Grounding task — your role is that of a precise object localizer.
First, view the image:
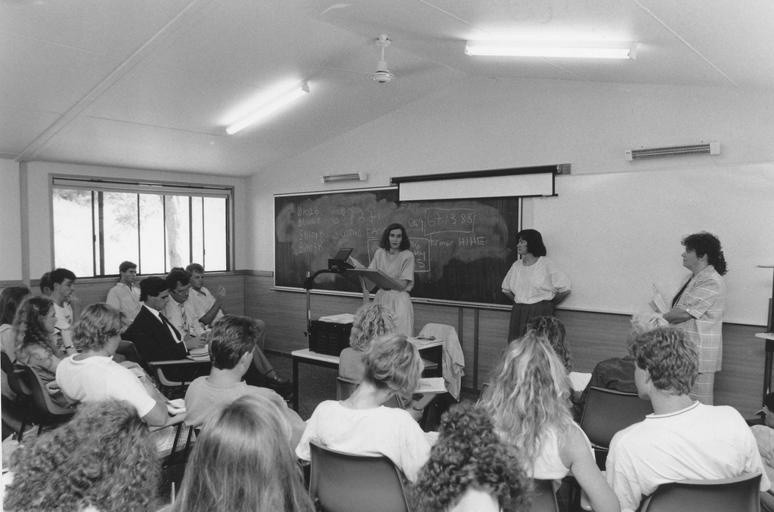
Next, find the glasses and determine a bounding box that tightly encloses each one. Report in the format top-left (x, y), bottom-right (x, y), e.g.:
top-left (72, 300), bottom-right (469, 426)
top-left (463, 45), bottom-right (637, 62)
top-left (321, 171), bottom-right (367, 184)
top-left (624, 141), bottom-right (721, 163)
top-left (225, 82), bottom-right (311, 136)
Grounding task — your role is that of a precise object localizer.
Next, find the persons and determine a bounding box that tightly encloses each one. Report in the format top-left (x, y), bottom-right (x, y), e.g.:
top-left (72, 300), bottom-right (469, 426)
top-left (1, 284), bottom-right (32, 403)
top-left (500, 228), bottom-right (572, 342)
top-left (296, 331), bottom-right (439, 487)
top-left (172, 394), bottom-right (317, 512)
top-left (185, 315), bottom-right (310, 451)
top-left (409, 400), bottom-right (542, 512)
top-left (40, 267), bottom-right (76, 347)
top-left (107, 262), bottom-right (142, 340)
top-left (476, 332), bottom-right (621, 511)
top-left (749, 394), bottom-right (773, 483)
top-left (663, 232), bottom-right (728, 406)
top-left (186, 264), bottom-right (224, 330)
top-left (56, 303), bottom-right (168, 427)
top-left (349, 222), bottom-right (417, 337)
top-left (605, 325), bottom-right (772, 512)
top-left (10, 295), bottom-right (79, 409)
top-left (124, 277), bottom-right (300, 398)
top-left (1, 395), bottom-right (162, 511)
top-left (577, 307), bottom-right (670, 422)
top-left (339, 303), bottom-right (424, 422)
top-left (522, 315), bottom-right (584, 423)
top-left (161, 266), bottom-right (296, 386)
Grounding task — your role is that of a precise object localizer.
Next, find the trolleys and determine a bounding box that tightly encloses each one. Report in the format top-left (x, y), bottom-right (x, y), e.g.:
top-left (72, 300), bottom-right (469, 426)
top-left (303, 34), bottom-right (449, 87)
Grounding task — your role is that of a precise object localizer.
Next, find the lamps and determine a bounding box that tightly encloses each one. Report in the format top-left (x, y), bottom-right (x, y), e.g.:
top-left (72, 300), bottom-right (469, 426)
top-left (321, 171), bottom-right (367, 184)
top-left (624, 141), bottom-right (721, 163)
top-left (225, 82), bottom-right (311, 136)
top-left (463, 45), bottom-right (637, 62)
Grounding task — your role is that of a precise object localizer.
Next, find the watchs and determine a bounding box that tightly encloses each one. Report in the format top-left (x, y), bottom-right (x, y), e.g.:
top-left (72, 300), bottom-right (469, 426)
top-left (65, 344), bottom-right (74, 349)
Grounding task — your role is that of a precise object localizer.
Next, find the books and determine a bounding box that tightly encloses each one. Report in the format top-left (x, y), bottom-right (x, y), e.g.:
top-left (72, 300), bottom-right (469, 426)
top-left (348, 268), bottom-right (405, 290)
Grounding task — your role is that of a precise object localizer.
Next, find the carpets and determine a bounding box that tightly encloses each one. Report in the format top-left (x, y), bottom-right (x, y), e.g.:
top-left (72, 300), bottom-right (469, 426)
top-left (159, 313), bottom-right (183, 341)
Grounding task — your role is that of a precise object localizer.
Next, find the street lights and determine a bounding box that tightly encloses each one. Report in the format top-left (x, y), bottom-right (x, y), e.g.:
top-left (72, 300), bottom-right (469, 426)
top-left (2, 414), bottom-right (46, 469)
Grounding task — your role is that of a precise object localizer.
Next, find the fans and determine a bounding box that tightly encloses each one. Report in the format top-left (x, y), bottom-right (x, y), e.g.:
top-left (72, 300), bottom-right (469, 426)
top-left (271, 184), bottom-right (523, 310)
top-left (303, 34), bottom-right (449, 87)
top-left (518, 163), bottom-right (772, 328)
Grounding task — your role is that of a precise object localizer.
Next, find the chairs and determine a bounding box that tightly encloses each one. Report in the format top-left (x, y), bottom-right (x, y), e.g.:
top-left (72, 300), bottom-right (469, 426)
top-left (0, 321), bottom-right (774, 511)
top-left (270, 374), bottom-right (290, 385)
